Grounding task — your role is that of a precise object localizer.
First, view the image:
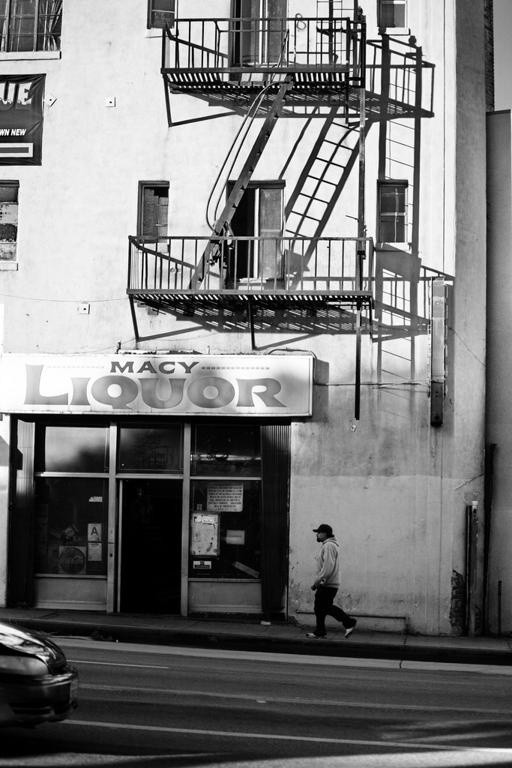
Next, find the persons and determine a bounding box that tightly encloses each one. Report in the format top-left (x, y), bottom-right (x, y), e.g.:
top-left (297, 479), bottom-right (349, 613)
top-left (305, 523), bottom-right (357, 640)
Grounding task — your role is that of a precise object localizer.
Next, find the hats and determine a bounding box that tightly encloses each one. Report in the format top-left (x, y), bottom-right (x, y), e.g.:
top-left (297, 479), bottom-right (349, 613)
top-left (1, 620), bottom-right (76, 728)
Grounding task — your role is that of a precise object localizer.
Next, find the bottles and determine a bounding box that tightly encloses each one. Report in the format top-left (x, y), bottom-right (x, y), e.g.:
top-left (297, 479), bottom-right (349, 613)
top-left (313, 525), bottom-right (332, 533)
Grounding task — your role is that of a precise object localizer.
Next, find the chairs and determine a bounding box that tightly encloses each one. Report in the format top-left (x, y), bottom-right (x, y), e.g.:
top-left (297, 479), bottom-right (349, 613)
top-left (344, 626), bottom-right (356, 638)
top-left (306, 632), bottom-right (327, 639)
